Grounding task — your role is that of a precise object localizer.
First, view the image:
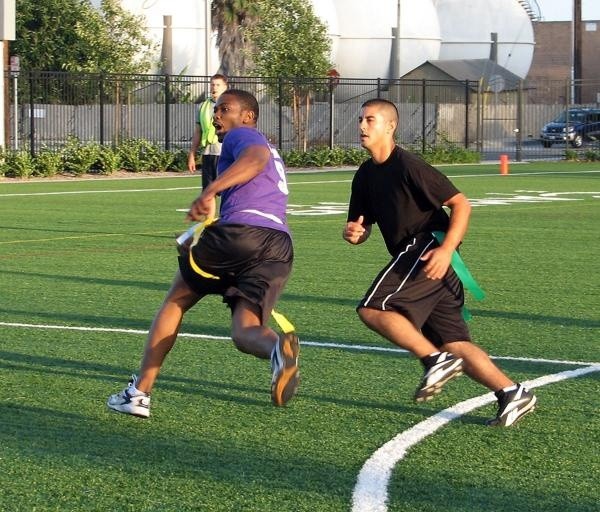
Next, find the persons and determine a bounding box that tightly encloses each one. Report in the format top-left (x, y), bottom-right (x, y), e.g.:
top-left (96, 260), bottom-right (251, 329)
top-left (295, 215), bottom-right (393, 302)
top-left (344, 98), bottom-right (540, 429)
top-left (185, 73), bottom-right (231, 219)
top-left (105, 89), bottom-right (303, 418)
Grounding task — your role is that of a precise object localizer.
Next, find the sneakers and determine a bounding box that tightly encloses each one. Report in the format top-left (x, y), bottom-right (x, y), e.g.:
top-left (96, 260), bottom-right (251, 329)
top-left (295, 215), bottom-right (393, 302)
top-left (487, 382), bottom-right (537, 426)
top-left (415, 351), bottom-right (463, 401)
top-left (270, 332), bottom-right (301, 407)
top-left (106, 388), bottom-right (151, 419)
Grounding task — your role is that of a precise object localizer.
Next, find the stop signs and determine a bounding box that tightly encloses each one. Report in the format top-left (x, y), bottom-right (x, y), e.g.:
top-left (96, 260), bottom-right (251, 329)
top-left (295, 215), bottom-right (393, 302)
top-left (325, 70), bottom-right (340, 89)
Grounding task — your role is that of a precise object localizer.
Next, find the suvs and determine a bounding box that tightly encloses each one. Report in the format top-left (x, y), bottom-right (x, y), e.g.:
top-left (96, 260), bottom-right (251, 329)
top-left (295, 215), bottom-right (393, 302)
top-left (540, 106), bottom-right (600, 149)
